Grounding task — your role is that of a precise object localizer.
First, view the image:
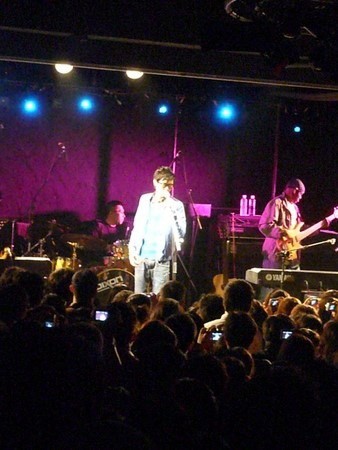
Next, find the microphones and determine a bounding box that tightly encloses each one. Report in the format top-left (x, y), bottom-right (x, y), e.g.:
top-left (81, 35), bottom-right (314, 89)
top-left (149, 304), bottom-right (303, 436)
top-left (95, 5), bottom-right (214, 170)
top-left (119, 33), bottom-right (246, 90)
top-left (221, 226), bottom-right (230, 241)
top-left (176, 150), bottom-right (182, 159)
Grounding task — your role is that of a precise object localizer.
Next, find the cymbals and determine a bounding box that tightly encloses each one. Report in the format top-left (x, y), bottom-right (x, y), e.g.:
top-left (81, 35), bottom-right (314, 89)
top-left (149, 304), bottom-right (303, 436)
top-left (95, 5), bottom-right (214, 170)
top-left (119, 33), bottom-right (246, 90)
top-left (43, 221), bottom-right (71, 232)
top-left (61, 232), bottom-right (107, 249)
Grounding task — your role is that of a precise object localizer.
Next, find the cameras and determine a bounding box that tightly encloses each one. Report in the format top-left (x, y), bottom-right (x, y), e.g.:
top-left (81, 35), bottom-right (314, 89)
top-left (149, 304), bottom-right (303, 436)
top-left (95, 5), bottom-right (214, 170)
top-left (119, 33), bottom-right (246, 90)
top-left (43, 321), bottom-right (55, 328)
top-left (209, 331), bottom-right (223, 341)
top-left (280, 330), bottom-right (292, 339)
top-left (94, 310), bottom-right (109, 321)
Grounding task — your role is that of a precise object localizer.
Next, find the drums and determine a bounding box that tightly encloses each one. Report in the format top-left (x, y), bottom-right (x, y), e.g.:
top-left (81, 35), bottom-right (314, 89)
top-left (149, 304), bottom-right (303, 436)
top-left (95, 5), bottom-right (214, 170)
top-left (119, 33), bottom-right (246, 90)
top-left (104, 256), bottom-right (134, 275)
top-left (88, 265), bottom-right (134, 306)
top-left (113, 239), bottom-right (130, 260)
top-left (51, 255), bottom-right (84, 274)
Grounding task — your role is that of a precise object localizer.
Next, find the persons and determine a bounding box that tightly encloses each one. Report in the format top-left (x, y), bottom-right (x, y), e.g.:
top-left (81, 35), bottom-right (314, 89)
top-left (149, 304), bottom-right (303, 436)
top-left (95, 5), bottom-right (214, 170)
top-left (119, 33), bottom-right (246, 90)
top-left (258, 179), bottom-right (306, 270)
top-left (127, 166), bottom-right (187, 294)
top-left (90, 200), bottom-right (133, 242)
top-left (0, 266), bottom-right (337, 450)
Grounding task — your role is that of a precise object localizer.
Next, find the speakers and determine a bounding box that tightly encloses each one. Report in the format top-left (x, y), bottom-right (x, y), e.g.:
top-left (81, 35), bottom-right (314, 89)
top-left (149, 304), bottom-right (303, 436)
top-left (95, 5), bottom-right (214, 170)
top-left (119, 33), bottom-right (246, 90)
top-left (245, 267), bottom-right (338, 302)
top-left (14, 256), bottom-right (52, 278)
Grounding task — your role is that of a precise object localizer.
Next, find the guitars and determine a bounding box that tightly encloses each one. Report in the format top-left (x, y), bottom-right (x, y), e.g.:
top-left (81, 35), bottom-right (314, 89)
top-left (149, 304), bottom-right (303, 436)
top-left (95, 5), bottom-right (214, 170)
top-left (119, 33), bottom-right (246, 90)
top-left (276, 206), bottom-right (338, 260)
top-left (212, 213), bottom-right (235, 295)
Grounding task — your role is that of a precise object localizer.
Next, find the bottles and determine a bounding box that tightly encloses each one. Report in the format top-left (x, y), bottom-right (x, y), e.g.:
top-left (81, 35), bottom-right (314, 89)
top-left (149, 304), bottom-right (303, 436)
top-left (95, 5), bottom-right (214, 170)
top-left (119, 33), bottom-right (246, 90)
top-left (248, 195), bottom-right (256, 217)
top-left (240, 195), bottom-right (248, 216)
